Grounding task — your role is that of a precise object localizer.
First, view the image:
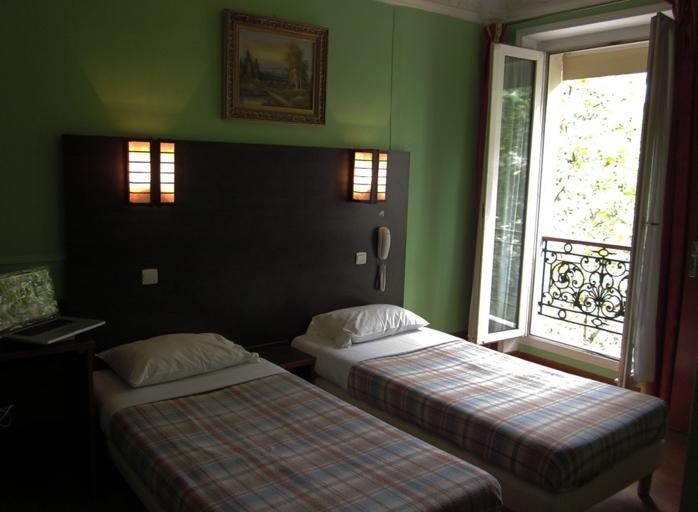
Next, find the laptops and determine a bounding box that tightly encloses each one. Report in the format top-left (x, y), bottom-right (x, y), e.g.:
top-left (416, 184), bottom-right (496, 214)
top-left (0, 265), bottom-right (108, 346)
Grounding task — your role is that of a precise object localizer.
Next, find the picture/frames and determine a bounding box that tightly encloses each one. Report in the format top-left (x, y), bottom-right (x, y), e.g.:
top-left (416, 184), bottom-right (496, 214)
top-left (220, 8), bottom-right (327, 127)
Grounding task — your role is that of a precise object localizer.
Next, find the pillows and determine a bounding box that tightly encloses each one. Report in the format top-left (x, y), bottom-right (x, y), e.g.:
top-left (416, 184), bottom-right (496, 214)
top-left (305, 303), bottom-right (431, 350)
top-left (94, 333), bottom-right (260, 389)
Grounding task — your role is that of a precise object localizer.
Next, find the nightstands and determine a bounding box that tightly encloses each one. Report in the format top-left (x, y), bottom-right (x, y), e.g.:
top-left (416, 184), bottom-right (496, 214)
top-left (0, 327), bottom-right (95, 433)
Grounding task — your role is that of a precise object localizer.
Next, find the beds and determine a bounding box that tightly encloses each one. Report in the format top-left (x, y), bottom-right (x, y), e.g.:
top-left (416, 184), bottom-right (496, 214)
top-left (84, 348), bottom-right (503, 512)
top-left (291, 313), bottom-right (669, 511)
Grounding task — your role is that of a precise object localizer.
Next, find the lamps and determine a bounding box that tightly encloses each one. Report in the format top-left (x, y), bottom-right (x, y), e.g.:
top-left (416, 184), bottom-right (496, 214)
top-left (121, 138), bottom-right (180, 209)
top-left (347, 148), bottom-right (388, 204)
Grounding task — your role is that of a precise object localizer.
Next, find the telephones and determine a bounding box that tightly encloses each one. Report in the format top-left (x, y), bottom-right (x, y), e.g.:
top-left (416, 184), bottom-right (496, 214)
top-left (378, 226), bottom-right (390, 293)
